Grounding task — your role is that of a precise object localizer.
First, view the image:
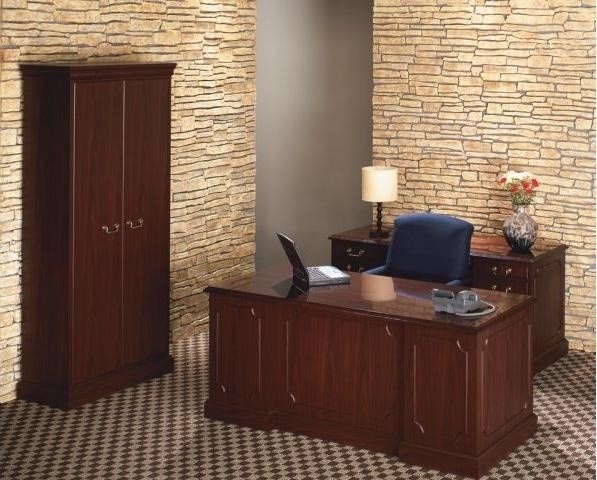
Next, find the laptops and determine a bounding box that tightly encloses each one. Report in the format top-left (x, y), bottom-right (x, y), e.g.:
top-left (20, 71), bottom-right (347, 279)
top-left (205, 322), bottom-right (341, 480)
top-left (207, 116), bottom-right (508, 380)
top-left (276, 232), bottom-right (351, 287)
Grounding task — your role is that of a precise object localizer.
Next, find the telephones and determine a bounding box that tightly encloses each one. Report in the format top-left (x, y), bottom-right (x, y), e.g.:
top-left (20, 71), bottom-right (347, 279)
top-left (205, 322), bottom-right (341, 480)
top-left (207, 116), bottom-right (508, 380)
top-left (432, 288), bottom-right (485, 314)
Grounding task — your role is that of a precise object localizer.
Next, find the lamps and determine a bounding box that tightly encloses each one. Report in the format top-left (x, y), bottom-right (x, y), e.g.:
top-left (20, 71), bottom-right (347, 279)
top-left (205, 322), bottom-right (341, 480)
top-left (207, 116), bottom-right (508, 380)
top-left (361, 157), bottom-right (400, 239)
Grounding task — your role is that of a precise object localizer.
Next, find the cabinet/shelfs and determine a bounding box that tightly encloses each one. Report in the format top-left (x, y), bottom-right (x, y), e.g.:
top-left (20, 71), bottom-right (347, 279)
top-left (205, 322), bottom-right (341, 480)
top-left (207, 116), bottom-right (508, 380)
top-left (15, 61), bottom-right (181, 412)
top-left (202, 266), bottom-right (540, 480)
top-left (327, 222), bottom-right (572, 375)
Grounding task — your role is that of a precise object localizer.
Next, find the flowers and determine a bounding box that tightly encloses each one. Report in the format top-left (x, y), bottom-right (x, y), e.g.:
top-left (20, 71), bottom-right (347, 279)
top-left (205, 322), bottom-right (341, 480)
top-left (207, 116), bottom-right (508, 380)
top-left (497, 170), bottom-right (541, 206)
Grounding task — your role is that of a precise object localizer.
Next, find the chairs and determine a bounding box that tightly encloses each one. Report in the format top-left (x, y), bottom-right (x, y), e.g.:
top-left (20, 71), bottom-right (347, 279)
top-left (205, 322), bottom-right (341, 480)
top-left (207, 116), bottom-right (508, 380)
top-left (360, 212), bottom-right (477, 286)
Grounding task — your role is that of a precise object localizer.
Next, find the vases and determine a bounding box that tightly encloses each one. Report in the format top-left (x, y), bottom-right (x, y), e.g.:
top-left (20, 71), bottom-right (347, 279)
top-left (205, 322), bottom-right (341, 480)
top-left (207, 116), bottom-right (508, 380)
top-left (501, 202), bottom-right (538, 253)
